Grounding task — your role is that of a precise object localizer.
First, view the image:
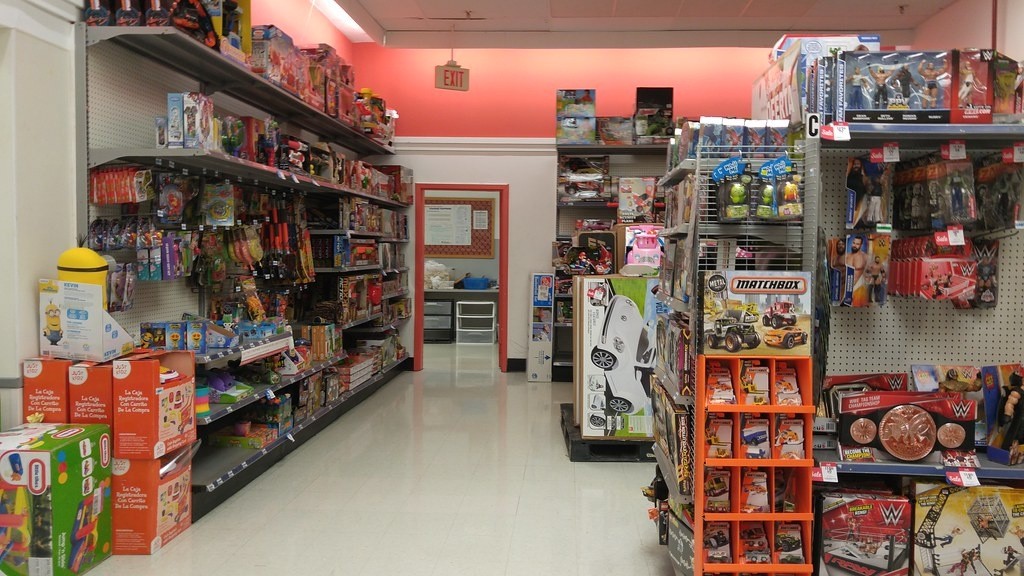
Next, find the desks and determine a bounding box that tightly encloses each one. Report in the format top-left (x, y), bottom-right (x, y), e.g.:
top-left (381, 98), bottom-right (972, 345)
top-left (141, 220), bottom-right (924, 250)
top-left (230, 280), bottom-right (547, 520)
top-left (423, 289), bottom-right (500, 345)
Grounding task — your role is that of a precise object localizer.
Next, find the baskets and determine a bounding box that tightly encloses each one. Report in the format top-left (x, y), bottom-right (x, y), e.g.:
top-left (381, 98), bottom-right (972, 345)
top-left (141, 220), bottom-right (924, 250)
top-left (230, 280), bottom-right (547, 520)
top-left (464, 278), bottom-right (488, 289)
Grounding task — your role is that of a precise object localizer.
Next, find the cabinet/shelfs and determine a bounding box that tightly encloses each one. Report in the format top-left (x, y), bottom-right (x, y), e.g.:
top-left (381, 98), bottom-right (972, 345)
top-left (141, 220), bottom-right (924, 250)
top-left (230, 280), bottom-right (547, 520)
top-left (548, 144), bottom-right (672, 379)
top-left (813, 123), bottom-right (1024, 576)
top-left (73, 21), bottom-right (411, 526)
top-left (651, 149), bottom-right (815, 575)
top-left (423, 299), bottom-right (496, 345)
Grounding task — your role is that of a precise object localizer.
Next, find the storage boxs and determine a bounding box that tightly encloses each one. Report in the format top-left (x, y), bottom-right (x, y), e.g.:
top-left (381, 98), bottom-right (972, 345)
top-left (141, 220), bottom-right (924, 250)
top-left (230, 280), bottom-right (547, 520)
top-left (558, 154), bottom-right (657, 222)
top-left (434, 281), bottom-right (455, 290)
top-left (649, 173), bottom-right (693, 498)
top-left (21, 346), bottom-right (198, 555)
top-left (166, 92), bottom-right (214, 149)
top-left (137, 164), bottom-right (413, 451)
top-left (250, 25), bottom-right (357, 127)
top-left (552, 87), bottom-right (679, 145)
top-left (768, 33), bottom-right (1024, 113)
top-left (239, 116), bottom-right (266, 163)
top-left (825, 361), bottom-right (1024, 466)
top-left (812, 476), bottom-right (1024, 576)
top-left (462, 278), bottom-right (488, 289)
top-left (569, 274), bottom-right (660, 442)
top-left (0, 424), bottom-right (116, 576)
